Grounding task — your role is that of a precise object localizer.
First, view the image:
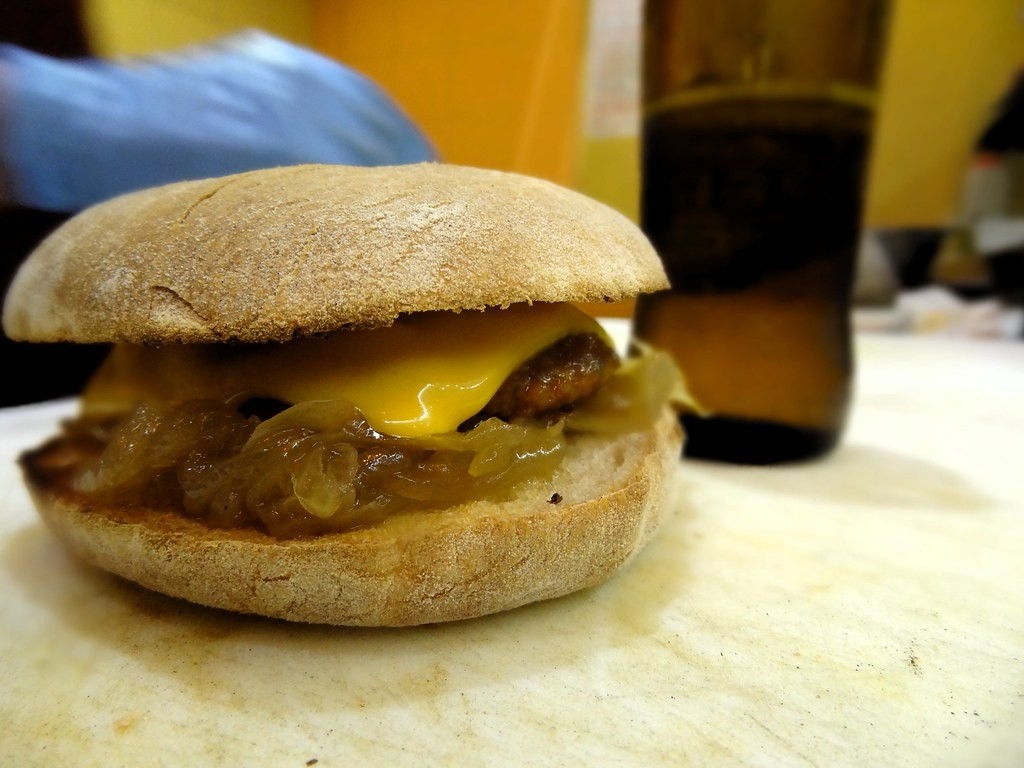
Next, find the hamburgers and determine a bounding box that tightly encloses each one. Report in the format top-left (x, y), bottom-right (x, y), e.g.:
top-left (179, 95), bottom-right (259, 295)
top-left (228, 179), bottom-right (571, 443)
top-left (3, 158), bottom-right (713, 627)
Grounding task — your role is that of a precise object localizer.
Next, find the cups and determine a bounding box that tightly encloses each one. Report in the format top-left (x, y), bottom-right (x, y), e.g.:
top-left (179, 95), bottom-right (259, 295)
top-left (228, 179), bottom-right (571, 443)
top-left (628, 0), bottom-right (895, 466)
